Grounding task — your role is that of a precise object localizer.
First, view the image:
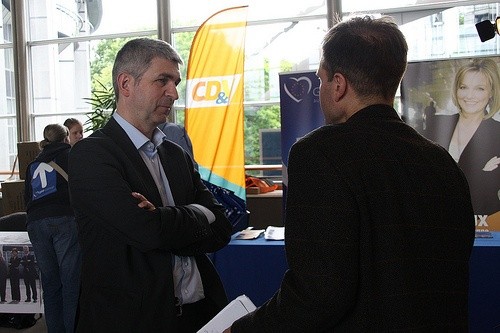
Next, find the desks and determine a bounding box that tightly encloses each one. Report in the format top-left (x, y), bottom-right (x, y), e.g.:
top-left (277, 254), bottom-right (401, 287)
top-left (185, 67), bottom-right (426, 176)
top-left (468, 230), bottom-right (500, 333)
top-left (206, 232), bottom-right (287, 304)
top-left (244, 190), bottom-right (285, 230)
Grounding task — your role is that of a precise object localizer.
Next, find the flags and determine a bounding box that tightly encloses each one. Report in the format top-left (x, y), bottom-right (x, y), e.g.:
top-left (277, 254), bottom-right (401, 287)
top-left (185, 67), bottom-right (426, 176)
top-left (186, 8), bottom-right (249, 205)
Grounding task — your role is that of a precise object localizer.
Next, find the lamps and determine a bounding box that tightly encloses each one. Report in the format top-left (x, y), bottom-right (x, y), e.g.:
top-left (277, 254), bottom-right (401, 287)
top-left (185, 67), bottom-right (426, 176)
top-left (474, 16), bottom-right (500, 43)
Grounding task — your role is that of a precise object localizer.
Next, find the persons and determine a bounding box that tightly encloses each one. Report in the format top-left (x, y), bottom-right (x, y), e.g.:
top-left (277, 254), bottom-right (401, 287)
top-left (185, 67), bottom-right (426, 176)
top-left (25, 118), bottom-right (83, 333)
top-left (221, 17), bottom-right (476, 333)
top-left (70, 37), bottom-right (232, 333)
top-left (0, 244), bottom-right (39, 305)
top-left (413, 58), bottom-right (500, 222)
